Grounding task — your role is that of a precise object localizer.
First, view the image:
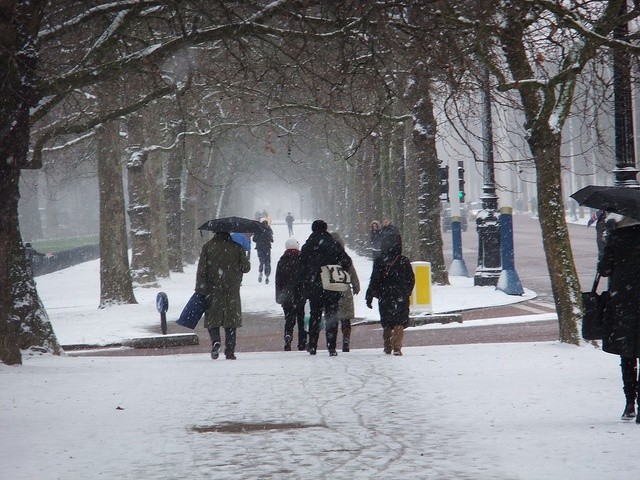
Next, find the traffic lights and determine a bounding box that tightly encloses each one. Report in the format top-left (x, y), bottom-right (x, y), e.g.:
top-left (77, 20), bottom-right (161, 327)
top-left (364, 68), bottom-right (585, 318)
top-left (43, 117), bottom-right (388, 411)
top-left (459, 166), bottom-right (465, 203)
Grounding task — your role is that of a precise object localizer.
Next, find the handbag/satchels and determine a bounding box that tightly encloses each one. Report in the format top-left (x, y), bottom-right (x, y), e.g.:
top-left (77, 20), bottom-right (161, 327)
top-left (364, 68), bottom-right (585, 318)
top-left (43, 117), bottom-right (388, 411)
top-left (175, 291), bottom-right (211, 330)
top-left (320, 264), bottom-right (351, 292)
top-left (582, 261), bottom-right (612, 339)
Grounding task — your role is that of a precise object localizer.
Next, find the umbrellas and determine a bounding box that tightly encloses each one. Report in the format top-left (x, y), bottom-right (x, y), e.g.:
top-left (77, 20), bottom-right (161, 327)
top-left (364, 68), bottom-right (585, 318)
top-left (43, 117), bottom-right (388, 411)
top-left (568, 185), bottom-right (640, 221)
top-left (196, 215), bottom-right (267, 238)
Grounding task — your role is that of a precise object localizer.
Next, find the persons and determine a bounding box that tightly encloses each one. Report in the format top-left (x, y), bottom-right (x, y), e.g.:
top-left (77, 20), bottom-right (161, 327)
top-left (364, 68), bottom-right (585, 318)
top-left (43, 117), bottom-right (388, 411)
top-left (195, 229), bottom-right (250, 360)
top-left (285, 212), bottom-right (295, 238)
top-left (364, 234), bottom-right (416, 356)
top-left (252, 220), bottom-right (274, 284)
top-left (230, 232), bottom-right (251, 286)
top-left (369, 220), bottom-right (382, 262)
top-left (330, 231), bottom-right (361, 352)
top-left (261, 209), bottom-right (269, 219)
top-left (580, 218), bottom-right (640, 424)
top-left (300, 219), bottom-right (352, 356)
top-left (254, 210), bottom-right (261, 220)
top-left (586, 209), bottom-right (610, 258)
top-left (373, 217), bottom-right (402, 254)
top-left (275, 238), bottom-right (308, 351)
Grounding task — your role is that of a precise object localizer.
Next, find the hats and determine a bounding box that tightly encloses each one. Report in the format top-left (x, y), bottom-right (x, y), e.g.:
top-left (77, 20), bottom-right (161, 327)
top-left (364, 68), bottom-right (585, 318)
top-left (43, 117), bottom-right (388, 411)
top-left (312, 220), bottom-right (327, 231)
top-left (285, 238), bottom-right (299, 249)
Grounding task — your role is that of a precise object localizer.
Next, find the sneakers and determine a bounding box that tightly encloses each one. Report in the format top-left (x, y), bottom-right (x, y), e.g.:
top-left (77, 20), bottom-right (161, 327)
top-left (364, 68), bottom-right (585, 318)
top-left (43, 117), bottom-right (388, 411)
top-left (393, 349), bottom-right (401, 355)
top-left (620, 404), bottom-right (636, 420)
top-left (211, 342), bottom-right (221, 359)
top-left (329, 349), bottom-right (336, 356)
top-left (283, 334), bottom-right (292, 351)
top-left (226, 351), bottom-right (237, 359)
top-left (258, 273), bottom-right (263, 282)
top-left (265, 280), bottom-right (270, 284)
